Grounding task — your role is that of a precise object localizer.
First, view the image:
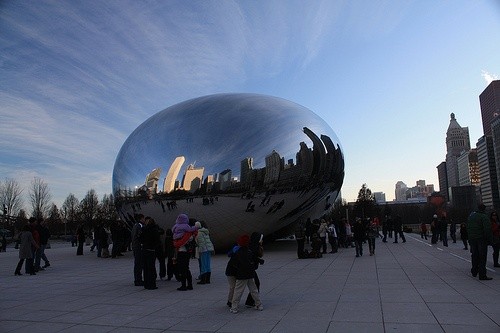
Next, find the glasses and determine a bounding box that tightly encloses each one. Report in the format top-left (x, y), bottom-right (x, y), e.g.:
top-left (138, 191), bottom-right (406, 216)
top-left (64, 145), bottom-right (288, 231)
top-left (492, 213), bottom-right (497, 216)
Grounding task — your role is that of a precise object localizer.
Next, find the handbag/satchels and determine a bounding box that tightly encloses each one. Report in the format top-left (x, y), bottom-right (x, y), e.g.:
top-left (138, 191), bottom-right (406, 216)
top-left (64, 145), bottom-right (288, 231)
top-left (101, 248), bottom-right (109, 257)
top-left (195, 246), bottom-right (199, 260)
top-left (431, 220), bottom-right (436, 228)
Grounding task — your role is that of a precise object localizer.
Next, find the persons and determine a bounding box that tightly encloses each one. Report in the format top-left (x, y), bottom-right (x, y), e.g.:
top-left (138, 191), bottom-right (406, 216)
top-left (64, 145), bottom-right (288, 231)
top-left (466, 203), bottom-right (500, 280)
top-left (420, 215), bottom-right (456, 247)
top-left (131, 212), bottom-right (214, 291)
top-left (240, 191), bottom-right (285, 215)
top-left (161, 200), bottom-right (176, 213)
top-left (203, 195), bottom-right (218, 205)
top-left (460, 223), bottom-right (468, 250)
top-left (186, 197), bottom-right (193, 203)
top-left (15, 215), bottom-right (50, 275)
top-left (295, 215), bottom-right (406, 258)
top-left (225, 231), bottom-right (265, 314)
top-left (71, 218), bottom-right (132, 258)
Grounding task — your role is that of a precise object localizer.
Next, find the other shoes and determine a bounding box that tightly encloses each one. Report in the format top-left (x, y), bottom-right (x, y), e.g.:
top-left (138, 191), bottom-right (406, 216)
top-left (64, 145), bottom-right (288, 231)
top-left (227, 302), bottom-right (232, 307)
top-left (402, 240), bottom-right (406, 243)
top-left (230, 309), bottom-right (237, 313)
top-left (135, 281), bottom-right (144, 286)
top-left (177, 287), bottom-right (185, 291)
top-left (330, 251), bottom-right (337, 253)
top-left (168, 276), bottom-right (171, 280)
top-left (393, 241), bottom-right (398, 243)
top-left (186, 286), bottom-right (193, 290)
top-left (245, 304), bottom-right (256, 308)
top-left (254, 305), bottom-right (263, 310)
top-left (14, 263), bottom-right (50, 276)
top-left (472, 273), bottom-right (477, 276)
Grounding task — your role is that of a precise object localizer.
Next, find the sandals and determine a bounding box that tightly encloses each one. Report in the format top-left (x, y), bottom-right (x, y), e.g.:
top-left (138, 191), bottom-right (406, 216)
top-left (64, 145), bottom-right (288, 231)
top-left (481, 277), bottom-right (492, 280)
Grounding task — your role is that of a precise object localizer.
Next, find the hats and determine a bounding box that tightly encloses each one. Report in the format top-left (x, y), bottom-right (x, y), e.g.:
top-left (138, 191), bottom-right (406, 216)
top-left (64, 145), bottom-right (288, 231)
top-left (239, 234), bottom-right (249, 244)
top-left (433, 214), bottom-right (437, 219)
top-left (195, 221), bottom-right (201, 228)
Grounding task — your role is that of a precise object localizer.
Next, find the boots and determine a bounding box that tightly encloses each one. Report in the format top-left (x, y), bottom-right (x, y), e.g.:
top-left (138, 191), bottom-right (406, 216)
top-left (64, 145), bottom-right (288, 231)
top-left (206, 272), bottom-right (211, 283)
top-left (321, 246), bottom-right (327, 253)
top-left (197, 273), bottom-right (206, 284)
top-left (493, 253), bottom-right (500, 267)
